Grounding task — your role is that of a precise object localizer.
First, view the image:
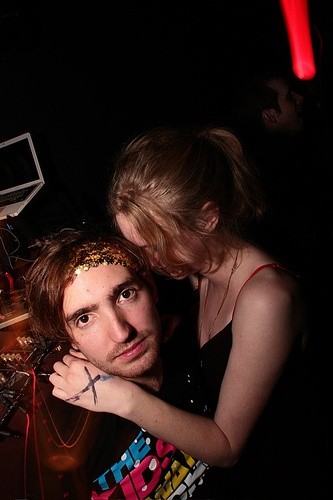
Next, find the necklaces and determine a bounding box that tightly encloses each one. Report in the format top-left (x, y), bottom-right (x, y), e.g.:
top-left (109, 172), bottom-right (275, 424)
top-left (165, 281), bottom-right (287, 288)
top-left (202, 241), bottom-right (237, 343)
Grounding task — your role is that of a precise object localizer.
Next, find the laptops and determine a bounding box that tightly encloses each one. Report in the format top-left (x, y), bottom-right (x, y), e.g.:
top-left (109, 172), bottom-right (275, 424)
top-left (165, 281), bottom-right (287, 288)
top-left (0, 134), bottom-right (46, 219)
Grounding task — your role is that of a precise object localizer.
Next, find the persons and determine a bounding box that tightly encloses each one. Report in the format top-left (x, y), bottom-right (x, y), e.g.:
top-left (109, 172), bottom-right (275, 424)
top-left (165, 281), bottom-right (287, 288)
top-left (41, 120), bottom-right (313, 500)
top-left (16, 229), bottom-right (218, 498)
